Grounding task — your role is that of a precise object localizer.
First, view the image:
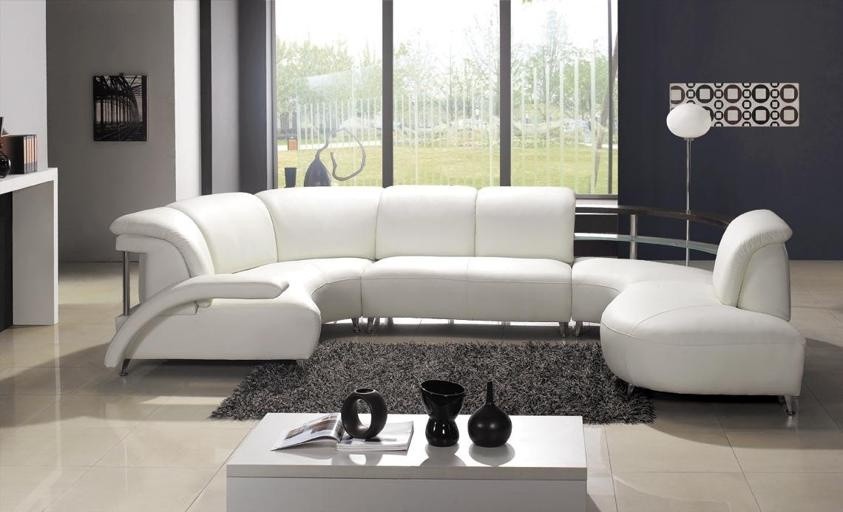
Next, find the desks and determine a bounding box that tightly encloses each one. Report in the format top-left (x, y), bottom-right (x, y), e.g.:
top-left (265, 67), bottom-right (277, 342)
top-left (0, 167), bottom-right (58, 331)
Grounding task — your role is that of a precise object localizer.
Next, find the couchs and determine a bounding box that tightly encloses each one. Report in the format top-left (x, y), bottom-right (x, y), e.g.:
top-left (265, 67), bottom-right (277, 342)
top-left (571, 209), bottom-right (807, 414)
top-left (103, 184), bottom-right (576, 378)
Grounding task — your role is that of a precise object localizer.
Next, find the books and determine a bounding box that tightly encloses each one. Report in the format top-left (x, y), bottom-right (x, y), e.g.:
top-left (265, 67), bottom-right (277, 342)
top-left (2, 133), bottom-right (38, 174)
top-left (271, 414), bottom-right (414, 451)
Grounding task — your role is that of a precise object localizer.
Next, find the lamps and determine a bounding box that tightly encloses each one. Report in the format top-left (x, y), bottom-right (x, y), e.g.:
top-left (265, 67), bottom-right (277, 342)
top-left (665, 103), bottom-right (711, 266)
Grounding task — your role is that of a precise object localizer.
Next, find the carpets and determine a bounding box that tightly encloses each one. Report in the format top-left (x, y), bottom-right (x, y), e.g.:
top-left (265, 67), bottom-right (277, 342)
top-left (208, 340), bottom-right (655, 424)
